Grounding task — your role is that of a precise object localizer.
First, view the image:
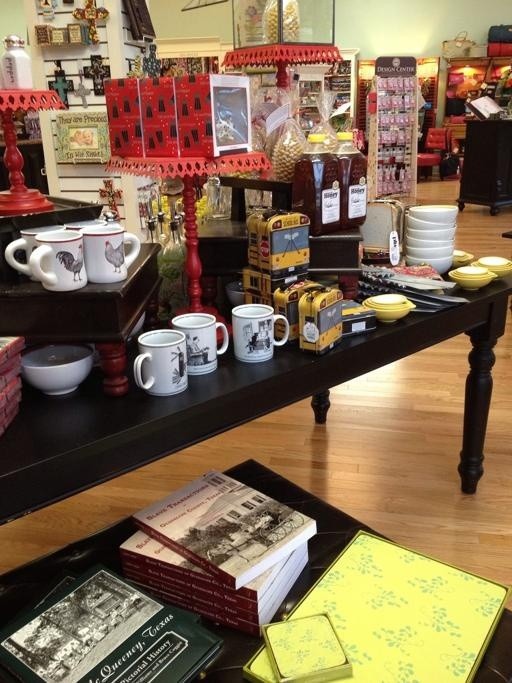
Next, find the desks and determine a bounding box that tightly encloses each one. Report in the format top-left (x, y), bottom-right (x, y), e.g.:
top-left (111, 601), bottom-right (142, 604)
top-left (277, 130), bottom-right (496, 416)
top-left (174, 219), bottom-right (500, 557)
top-left (0, 263), bottom-right (510, 523)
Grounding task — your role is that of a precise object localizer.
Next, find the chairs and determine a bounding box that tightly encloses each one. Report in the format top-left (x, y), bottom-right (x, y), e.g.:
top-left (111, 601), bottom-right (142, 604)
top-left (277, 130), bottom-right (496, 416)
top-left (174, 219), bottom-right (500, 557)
top-left (418, 125), bottom-right (452, 182)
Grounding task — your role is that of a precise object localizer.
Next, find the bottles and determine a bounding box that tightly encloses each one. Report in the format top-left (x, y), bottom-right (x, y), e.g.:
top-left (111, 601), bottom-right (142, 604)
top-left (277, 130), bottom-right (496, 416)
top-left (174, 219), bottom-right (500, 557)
top-left (144, 212), bottom-right (190, 319)
top-left (332, 132), bottom-right (366, 228)
top-left (2, 34), bottom-right (33, 90)
top-left (291, 133), bottom-right (341, 236)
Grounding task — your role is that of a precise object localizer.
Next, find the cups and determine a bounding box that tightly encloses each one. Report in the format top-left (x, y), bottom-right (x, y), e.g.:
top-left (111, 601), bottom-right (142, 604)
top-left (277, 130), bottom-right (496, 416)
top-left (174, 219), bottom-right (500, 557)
top-left (134, 327), bottom-right (191, 397)
top-left (171, 311), bottom-right (231, 377)
top-left (4, 220), bottom-right (141, 291)
top-left (232, 303), bottom-right (291, 365)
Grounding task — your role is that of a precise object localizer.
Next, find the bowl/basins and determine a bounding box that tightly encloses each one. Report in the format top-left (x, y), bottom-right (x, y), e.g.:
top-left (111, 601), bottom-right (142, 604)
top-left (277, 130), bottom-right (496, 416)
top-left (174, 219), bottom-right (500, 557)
top-left (451, 249), bottom-right (512, 293)
top-left (406, 204), bottom-right (459, 274)
top-left (364, 294), bottom-right (416, 322)
top-left (21, 344), bottom-right (94, 397)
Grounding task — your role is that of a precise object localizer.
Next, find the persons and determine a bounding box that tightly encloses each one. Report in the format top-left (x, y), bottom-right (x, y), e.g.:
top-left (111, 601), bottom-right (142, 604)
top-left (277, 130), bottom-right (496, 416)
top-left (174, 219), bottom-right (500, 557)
top-left (70, 130), bottom-right (84, 149)
top-left (82, 129), bottom-right (98, 148)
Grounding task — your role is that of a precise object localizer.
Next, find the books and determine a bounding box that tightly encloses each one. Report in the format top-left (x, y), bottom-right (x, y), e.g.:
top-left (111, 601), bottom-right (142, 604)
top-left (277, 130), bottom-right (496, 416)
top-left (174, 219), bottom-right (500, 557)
top-left (119, 467), bottom-right (319, 639)
top-left (0, 569), bottom-right (227, 683)
